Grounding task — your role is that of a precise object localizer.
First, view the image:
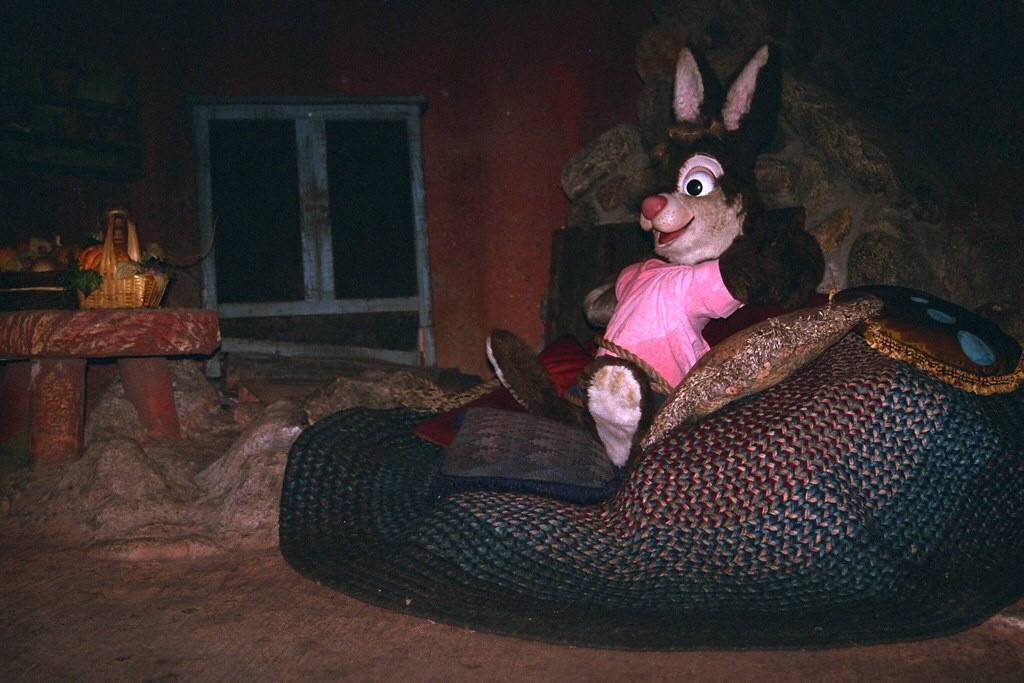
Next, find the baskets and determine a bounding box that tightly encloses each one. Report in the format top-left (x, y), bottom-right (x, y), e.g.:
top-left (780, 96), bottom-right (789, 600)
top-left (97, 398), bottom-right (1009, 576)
top-left (76, 211), bottom-right (167, 309)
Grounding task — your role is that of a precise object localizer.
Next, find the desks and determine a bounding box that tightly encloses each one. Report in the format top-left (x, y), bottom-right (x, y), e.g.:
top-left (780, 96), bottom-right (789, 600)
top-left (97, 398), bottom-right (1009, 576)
top-left (0, 307), bottom-right (220, 471)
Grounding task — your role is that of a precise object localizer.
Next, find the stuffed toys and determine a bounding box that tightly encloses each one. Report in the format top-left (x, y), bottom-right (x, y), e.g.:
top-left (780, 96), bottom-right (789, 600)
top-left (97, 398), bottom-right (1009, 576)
top-left (484, 44), bottom-right (825, 474)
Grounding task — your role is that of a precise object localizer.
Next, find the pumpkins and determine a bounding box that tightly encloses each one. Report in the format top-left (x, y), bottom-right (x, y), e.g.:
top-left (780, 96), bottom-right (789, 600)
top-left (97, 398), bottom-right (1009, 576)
top-left (78, 244), bottom-right (133, 276)
